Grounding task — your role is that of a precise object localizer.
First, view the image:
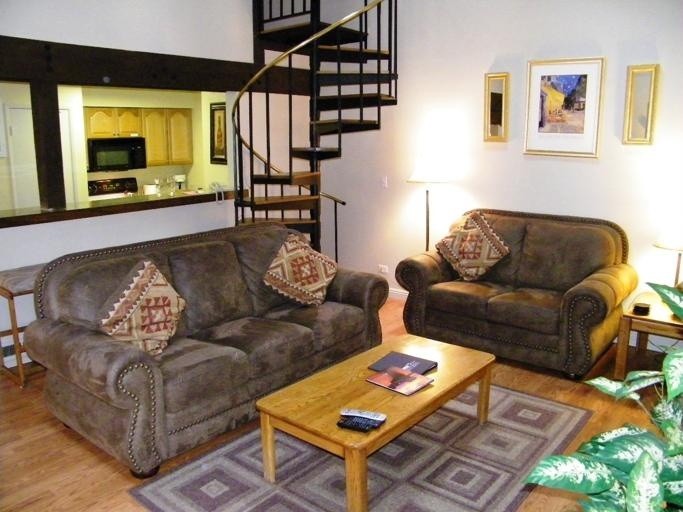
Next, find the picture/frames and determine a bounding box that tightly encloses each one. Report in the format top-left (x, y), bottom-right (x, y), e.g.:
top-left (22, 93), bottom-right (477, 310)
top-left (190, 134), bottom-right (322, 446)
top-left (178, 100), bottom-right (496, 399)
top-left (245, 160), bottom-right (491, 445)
top-left (521, 59), bottom-right (606, 158)
top-left (209, 102), bottom-right (227, 164)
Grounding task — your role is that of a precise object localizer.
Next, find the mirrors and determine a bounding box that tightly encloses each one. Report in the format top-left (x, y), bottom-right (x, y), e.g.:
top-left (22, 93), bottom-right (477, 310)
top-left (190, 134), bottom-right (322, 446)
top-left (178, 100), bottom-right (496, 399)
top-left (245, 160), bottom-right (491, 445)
top-left (486, 72), bottom-right (508, 143)
top-left (623, 65), bottom-right (661, 144)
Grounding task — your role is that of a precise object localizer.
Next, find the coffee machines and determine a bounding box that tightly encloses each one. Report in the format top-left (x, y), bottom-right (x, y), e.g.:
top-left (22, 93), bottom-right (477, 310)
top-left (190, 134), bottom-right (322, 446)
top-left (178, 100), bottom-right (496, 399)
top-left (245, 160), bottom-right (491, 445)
top-left (174, 175), bottom-right (185, 190)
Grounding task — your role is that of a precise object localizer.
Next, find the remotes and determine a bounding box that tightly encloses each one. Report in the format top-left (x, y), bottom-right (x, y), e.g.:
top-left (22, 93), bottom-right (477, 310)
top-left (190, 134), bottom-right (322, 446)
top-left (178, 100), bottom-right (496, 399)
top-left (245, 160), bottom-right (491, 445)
top-left (340, 408), bottom-right (385, 422)
top-left (337, 419), bottom-right (369, 432)
top-left (346, 417), bottom-right (379, 426)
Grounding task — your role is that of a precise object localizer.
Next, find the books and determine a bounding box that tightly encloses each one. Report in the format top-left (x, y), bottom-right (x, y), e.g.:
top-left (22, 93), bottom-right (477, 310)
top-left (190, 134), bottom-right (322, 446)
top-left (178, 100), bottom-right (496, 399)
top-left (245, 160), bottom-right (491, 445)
top-left (368, 365), bottom-right (435, 395)
top-left (368, 350), bottom-right (437, 375)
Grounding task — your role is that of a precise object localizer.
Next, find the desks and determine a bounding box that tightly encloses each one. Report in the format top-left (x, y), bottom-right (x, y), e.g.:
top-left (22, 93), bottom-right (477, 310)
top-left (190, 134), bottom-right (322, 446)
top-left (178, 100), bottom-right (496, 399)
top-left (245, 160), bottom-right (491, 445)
top-left (0, 264), bottom-right (55, 388)
top-left (613, 292), bottom-right (683, 381)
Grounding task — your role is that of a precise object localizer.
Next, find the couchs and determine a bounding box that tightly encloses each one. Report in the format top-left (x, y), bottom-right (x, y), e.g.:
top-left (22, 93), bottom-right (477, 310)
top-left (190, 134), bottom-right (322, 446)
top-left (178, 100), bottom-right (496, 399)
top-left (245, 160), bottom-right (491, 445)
top-left (21, 221), bottom-right (390, 479)
top-left (393, 209), bottom-right (639, 382)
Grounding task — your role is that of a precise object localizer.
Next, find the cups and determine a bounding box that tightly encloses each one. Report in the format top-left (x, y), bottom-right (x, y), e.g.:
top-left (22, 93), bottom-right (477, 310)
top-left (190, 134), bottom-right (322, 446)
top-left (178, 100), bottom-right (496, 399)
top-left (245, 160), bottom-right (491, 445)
top-left (155, 175), bottom-right (163, 197)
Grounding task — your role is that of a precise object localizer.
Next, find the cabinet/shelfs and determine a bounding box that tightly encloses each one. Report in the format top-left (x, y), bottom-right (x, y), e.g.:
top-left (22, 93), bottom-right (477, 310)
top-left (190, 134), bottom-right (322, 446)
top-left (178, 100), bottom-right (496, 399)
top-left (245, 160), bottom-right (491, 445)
top-left (83, 105), bottom-right (195, 165)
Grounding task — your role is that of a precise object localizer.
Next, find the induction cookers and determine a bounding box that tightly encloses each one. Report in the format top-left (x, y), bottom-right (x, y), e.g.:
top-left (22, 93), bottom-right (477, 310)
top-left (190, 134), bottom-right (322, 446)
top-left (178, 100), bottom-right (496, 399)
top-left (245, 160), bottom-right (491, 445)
top-left (88, 177), bottom-right (138, 202)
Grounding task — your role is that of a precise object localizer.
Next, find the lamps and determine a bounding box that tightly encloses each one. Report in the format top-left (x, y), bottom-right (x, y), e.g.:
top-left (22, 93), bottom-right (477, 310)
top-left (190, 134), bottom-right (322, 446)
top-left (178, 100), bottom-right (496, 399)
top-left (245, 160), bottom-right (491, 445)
top-left (408, 155), bottom-right (449, 251)
top-left (652, 234), bottom-right (682, 304)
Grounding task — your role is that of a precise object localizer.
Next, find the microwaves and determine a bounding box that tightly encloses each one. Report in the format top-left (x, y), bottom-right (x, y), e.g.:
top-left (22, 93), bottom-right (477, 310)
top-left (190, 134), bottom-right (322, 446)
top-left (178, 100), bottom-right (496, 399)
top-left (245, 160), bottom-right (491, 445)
top-left (86, 137), bottom-right (147, 173)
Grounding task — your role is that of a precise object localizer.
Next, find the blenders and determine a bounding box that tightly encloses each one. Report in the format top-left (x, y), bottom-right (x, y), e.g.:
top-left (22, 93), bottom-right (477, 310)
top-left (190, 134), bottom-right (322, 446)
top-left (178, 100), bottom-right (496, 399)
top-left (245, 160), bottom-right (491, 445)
top-left (142, 184), bottom-right (156, 195)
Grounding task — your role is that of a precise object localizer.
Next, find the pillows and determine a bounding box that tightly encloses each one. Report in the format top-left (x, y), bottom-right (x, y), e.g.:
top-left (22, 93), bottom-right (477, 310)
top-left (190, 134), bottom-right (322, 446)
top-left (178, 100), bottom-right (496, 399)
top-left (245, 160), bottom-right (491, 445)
top-left (96, 259), bottom-right (189, 359)
top-left (264, 234), bottom-right (340, 306)
top-left (434, 211), bottom-right (507, 282)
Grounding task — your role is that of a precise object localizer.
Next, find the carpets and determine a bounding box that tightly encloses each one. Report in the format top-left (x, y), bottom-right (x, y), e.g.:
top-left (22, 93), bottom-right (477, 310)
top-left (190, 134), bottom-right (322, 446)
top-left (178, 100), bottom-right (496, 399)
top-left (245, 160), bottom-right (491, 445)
top-left (128, 382), bottom-right (595, 512)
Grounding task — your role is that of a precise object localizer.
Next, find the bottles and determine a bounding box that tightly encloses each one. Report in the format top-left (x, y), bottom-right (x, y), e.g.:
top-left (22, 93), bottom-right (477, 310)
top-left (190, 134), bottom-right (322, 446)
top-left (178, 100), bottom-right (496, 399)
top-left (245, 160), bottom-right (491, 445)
top-left (217, 116), bottom-right (223, 150)
top-left (167, 175), bottom-right (176, 196)
